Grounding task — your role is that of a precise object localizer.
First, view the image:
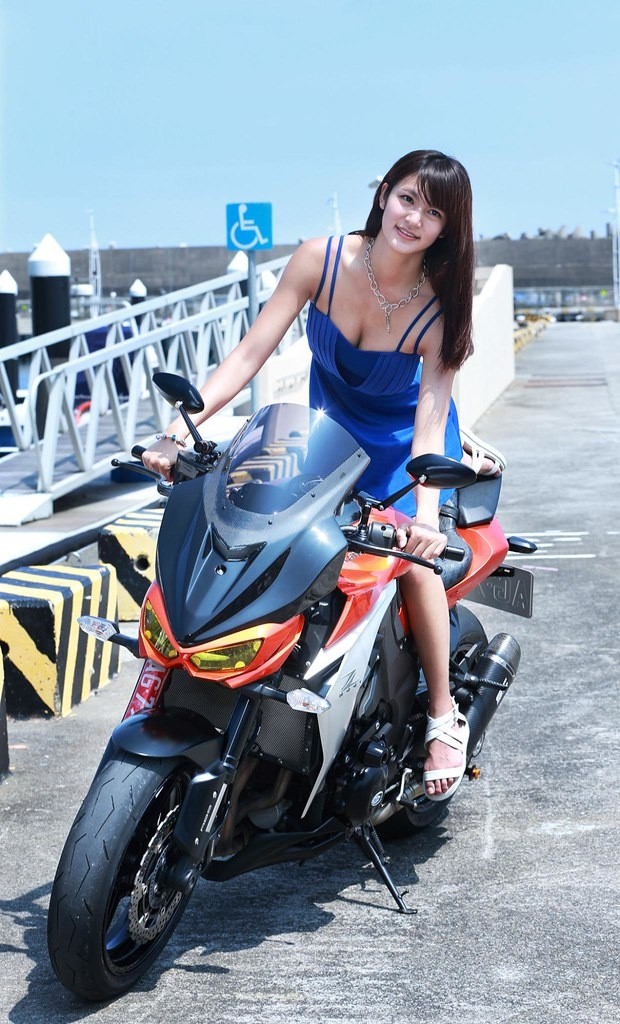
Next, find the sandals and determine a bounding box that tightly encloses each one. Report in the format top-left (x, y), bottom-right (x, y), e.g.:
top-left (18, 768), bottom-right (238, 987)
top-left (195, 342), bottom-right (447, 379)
top-left (459, 424), bottom-right (507, 477)
top-left (422, 696), bottom-right (470, 802)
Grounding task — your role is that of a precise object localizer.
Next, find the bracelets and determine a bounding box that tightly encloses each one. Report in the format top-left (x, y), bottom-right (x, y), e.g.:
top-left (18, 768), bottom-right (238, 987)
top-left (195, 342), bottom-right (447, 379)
top-left (155, 434), bottom-right (186, 448)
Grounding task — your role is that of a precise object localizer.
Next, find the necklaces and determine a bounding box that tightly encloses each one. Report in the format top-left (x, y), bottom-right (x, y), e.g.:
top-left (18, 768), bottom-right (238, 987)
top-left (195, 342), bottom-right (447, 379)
top-left (364, 236), bottom-right (428, 334)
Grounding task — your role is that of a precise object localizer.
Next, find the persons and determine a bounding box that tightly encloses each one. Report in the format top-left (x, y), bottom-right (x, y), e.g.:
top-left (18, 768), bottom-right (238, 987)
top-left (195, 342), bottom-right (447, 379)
top-left (140, 148), bottom-right (507, 802)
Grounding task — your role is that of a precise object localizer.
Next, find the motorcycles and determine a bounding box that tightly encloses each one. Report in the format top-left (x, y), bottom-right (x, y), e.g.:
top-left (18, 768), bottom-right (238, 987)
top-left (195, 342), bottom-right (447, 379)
top-left (46, 371), bottom-right (537, 1001)
